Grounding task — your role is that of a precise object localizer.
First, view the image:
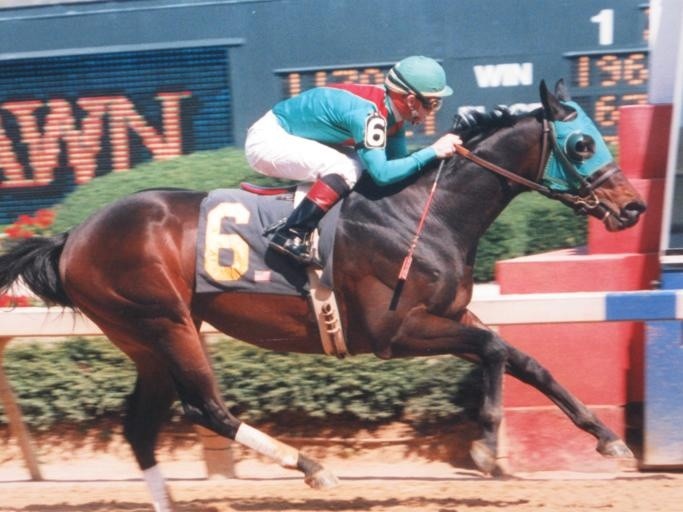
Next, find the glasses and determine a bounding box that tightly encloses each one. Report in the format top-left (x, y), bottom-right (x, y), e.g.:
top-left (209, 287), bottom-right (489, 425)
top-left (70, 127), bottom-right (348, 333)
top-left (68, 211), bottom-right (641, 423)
top-left (387, 64), bottom-right (442, 114)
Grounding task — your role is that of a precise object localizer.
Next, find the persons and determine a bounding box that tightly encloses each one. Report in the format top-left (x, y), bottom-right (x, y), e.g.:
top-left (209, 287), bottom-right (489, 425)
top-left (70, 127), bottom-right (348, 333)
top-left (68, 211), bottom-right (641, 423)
top-left (245, 57), bottom-right (463, 269)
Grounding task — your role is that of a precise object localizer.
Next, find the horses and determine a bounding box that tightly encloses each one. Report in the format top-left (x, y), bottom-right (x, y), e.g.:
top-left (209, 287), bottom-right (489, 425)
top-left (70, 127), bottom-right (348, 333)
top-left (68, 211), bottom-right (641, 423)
top-left (0, 79), bottom-right (646, 512)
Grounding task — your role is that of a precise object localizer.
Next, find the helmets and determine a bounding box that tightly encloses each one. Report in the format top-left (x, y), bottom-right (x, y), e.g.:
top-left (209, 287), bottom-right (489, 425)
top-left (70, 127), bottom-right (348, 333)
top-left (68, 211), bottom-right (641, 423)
top-left (385, 56), bottom-right (455, 98)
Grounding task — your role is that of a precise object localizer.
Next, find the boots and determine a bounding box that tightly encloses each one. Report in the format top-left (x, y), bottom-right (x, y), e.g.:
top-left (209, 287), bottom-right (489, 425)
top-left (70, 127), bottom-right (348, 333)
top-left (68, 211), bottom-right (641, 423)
top-left (268, 173), bottom-right (351, 269)
